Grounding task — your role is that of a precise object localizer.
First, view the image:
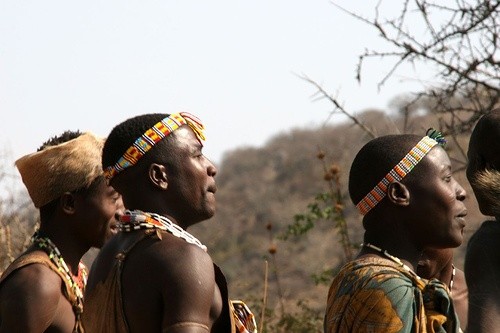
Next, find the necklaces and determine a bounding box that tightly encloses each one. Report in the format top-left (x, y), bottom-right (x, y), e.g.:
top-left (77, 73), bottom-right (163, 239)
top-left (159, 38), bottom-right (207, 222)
top-left (360, 243), bottom-right (456, 295)
top-left (111, 210), bottom-right (208, 252)
top-left (29, 228), bottom-right (88, 313)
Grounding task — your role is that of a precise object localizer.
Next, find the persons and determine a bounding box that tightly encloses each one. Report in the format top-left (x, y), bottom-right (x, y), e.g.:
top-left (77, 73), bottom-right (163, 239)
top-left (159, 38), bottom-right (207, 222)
top-left (464, 112), bottom-right (500, 333)
top-left (83, 114), bottom-right (258, 333)
top-left (0, 132), bottom-right (128, 333)
top-left (323, 128), bottom-right (467, 333)
